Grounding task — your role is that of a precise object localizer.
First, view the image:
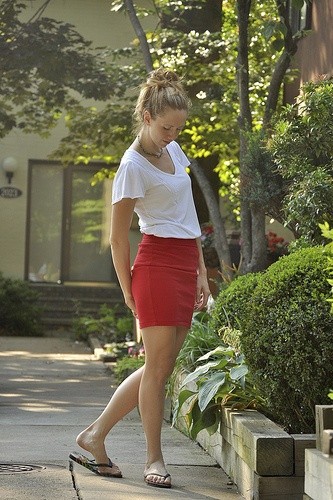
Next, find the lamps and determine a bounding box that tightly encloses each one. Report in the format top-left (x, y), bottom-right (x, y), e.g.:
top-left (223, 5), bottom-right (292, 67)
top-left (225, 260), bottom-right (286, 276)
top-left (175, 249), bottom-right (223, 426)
top-left (3, 157), bottom-right (19, 184)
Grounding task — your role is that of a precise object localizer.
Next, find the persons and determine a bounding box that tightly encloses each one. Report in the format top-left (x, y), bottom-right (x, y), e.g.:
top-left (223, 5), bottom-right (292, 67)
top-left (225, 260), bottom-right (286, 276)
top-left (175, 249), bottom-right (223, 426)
top-left (70, 70), bottom-right (210, 487)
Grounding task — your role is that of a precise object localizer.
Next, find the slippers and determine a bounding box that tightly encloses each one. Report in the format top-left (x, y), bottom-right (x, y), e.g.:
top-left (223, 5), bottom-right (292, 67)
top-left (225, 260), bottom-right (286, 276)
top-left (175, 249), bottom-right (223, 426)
top-left (143, 473), bottom-right (171, 488)
top-left (68, 450), bottom-right (122, 478)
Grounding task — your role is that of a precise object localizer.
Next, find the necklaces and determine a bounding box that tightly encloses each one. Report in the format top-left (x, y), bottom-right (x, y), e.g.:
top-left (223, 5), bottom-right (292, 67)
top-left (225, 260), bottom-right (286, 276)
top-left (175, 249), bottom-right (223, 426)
top-left (137, 135), bottom-right (163, 158)
